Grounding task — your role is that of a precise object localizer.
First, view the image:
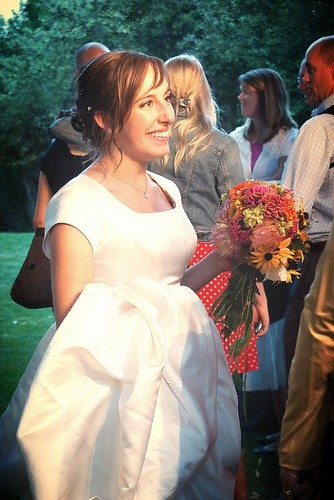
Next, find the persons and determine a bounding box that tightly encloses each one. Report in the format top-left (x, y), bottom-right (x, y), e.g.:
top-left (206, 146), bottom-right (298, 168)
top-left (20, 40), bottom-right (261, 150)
top-left (42, 49), bottom-right (269, 500)
top-left (32, 36), bottom-right (334, 500)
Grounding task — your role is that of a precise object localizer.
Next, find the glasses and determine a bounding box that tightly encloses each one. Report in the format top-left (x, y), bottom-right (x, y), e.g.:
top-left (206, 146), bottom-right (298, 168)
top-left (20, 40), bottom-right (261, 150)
top-left (297, 76), bottom-right (303, 82)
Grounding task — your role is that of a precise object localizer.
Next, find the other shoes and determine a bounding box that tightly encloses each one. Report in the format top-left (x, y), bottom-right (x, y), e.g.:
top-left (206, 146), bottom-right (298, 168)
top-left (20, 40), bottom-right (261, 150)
top-left (255, 436), bottom-right (272, 444)
top-left (252, 445), bottom-right (278, 455)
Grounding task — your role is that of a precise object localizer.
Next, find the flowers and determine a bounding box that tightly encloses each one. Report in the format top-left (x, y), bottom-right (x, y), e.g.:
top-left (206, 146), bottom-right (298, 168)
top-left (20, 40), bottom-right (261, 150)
top-left (209, 180), bottom-right (321, 392)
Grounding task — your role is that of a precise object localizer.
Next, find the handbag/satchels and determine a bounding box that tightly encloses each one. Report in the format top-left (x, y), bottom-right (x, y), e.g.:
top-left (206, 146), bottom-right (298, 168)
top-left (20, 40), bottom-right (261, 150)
top-left (11, 228), bottom-right (53, 310)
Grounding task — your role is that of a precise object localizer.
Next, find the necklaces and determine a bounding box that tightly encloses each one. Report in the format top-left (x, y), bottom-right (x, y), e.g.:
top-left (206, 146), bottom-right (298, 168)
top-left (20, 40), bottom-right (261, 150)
top-left (96, 157), bottom-right (149, 199)
